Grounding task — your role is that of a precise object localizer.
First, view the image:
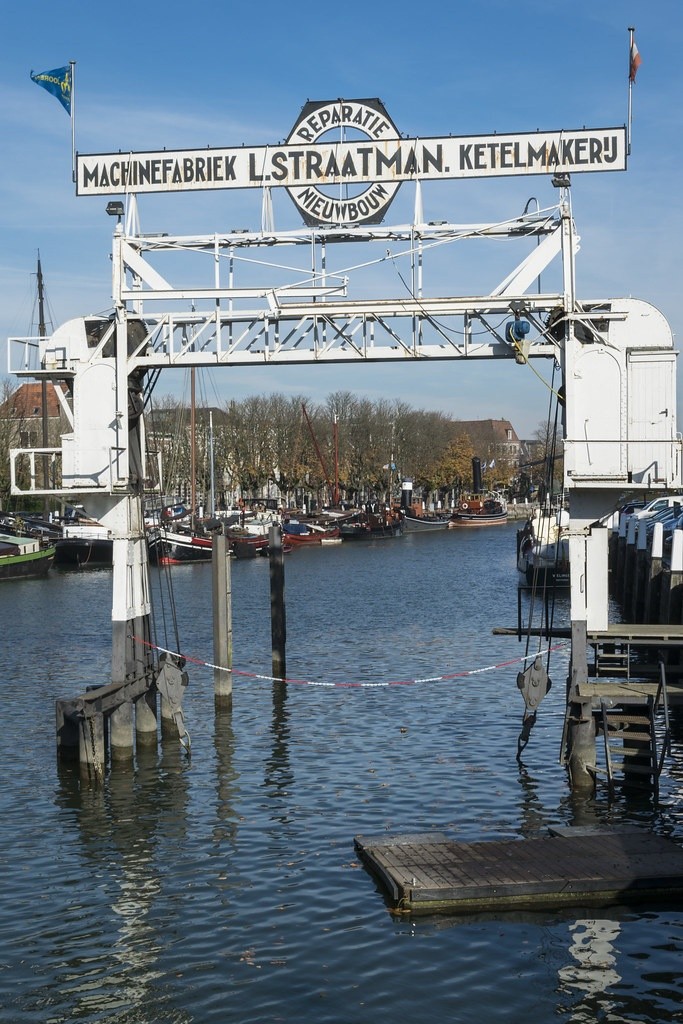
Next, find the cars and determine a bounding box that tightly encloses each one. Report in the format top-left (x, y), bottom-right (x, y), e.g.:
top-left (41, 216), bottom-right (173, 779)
top-left (618, 496), bottom-right (683, 547)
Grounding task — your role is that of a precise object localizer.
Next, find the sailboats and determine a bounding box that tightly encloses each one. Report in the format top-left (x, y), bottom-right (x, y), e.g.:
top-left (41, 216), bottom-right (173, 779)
top-left (0, 247), bottom-right (508, 579)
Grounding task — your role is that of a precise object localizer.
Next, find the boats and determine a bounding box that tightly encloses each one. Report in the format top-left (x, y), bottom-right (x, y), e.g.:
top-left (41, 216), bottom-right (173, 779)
top-left (517, 492), bottom-right (613, 590)
